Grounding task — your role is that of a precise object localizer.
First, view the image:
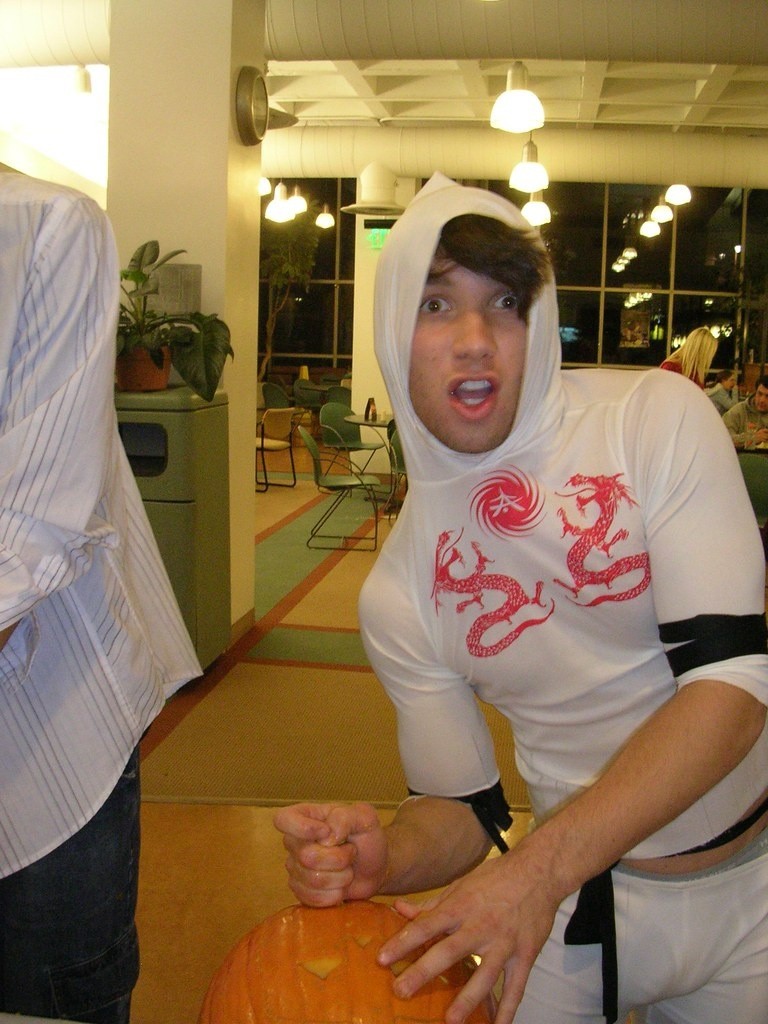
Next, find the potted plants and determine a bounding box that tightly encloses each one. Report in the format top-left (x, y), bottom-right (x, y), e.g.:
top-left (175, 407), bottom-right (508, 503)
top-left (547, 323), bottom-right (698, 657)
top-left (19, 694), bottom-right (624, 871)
top-left (113, 240), bottom-right (236, 404)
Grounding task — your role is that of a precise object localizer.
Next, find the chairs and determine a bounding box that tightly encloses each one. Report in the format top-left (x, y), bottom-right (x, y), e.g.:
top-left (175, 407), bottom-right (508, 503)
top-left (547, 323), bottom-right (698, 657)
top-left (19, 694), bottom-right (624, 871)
top-left (319, 402), bottom-right (397, 503)
top-left (261, 371), bottom-right (352, 448)
top-left (383, 419), bottom-right (409, 524)
top-left (255, 407), bottom-right (297, 493)
top-left (298, 425), bottom-right (382, 552)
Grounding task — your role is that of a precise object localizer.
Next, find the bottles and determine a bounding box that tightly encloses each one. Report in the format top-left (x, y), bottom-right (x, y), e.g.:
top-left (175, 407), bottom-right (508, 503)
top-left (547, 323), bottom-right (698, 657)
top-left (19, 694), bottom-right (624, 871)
top-left (364, 397), bottom-right (377, 420)
top-left (744, 423), bottom-right (755, 450)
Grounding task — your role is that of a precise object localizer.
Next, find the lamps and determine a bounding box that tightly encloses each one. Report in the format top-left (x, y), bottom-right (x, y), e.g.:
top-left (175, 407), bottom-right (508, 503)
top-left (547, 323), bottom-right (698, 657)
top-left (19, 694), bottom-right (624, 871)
top-left (260, 59), bottom-right (693, 240)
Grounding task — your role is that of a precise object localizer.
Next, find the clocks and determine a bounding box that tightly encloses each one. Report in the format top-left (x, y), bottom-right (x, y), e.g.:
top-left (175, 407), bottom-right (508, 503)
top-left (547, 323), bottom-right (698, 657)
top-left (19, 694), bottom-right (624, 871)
top-left (236, 65), bottom-right (270, 147)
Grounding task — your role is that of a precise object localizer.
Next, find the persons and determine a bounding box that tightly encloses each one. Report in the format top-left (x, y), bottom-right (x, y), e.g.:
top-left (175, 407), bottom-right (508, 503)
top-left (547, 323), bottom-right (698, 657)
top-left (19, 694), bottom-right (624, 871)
top-left (0, 156), bottom-right (204, 1024)
top-left (705, 369), bottom-right (768, 449)
top-left (659, 327), bottom-right (718, 391)
top-left (273, 185), bottom-right (768, 1024)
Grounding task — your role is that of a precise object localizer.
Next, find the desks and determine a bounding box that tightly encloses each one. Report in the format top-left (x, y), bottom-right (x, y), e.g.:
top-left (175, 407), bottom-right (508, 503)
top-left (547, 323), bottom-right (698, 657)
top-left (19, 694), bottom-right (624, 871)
top-left (299, 383), bottom-right (352, 442)
top-left (344, 413), bottom-right (409, 513)
